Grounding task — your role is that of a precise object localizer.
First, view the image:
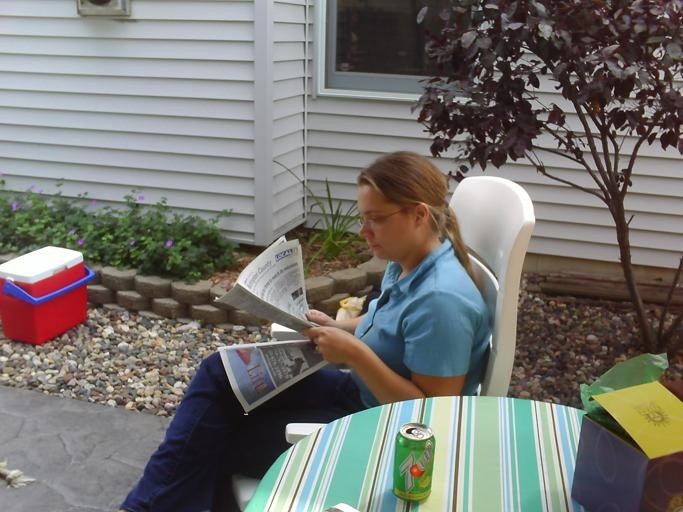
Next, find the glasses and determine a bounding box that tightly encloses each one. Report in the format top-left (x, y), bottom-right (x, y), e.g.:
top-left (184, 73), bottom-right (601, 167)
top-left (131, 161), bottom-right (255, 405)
top-left (358, 204), bottom-right (418, 229)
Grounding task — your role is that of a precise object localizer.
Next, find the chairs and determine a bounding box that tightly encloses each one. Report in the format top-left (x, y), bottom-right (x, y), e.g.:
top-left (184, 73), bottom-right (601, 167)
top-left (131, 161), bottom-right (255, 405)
top-left (233, 177), bottom-right (536, 510)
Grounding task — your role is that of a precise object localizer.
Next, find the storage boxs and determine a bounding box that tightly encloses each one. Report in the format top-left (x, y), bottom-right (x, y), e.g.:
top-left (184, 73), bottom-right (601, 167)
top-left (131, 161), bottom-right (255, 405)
top-left (571, 379), bottom-right (683, 510)
top-left (0, 246), bottom-right (95, 345)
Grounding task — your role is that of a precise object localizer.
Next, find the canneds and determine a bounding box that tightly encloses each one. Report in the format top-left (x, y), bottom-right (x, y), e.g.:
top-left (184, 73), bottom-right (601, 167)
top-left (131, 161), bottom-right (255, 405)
top-left (392, 420), bottom-right (435, 503)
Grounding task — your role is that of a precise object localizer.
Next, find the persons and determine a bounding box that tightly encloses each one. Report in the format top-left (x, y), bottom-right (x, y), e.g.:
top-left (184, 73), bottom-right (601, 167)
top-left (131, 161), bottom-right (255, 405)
top-left (120, 149), bottom-right (492, 511)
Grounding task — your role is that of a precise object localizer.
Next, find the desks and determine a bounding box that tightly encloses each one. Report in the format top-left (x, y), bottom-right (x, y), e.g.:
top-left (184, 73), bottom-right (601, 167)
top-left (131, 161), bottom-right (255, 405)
top-left (243, 396), bottom-right (587, 512)
